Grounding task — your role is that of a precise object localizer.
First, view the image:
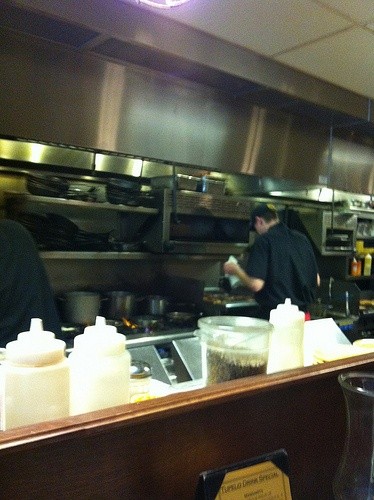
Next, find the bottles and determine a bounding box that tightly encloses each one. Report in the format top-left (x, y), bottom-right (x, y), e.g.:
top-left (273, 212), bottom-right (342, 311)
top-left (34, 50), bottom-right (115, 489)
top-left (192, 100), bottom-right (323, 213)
top-left (68, 315), bottom-right (131, 416)
top-left (364, 253), bottom-right (372, 276)
top-left (333, 371), bottom-right (374, 500)
top-left (1, 318), bottom-right (70, 432)
top-left (351, 257), bottom-right (362, 277)
top-left (266, 298), bottom-right (305, 374)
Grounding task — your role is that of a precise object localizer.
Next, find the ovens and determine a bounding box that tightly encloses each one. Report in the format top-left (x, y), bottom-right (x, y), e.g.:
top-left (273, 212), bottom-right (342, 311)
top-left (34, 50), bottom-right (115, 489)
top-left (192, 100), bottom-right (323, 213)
top-left (150, 190), bottom-right (287, 255)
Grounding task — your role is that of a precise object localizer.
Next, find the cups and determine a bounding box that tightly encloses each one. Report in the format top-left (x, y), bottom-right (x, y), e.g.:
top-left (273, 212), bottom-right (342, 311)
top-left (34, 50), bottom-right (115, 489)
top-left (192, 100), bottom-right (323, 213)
top-left (199, 315), bottom-right (275, 387)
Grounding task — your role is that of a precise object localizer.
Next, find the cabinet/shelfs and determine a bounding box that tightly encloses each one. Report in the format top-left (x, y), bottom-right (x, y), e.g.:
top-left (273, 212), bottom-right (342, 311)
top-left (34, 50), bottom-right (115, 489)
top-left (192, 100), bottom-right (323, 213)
top-left (0, 189), bottom-right (160, 260)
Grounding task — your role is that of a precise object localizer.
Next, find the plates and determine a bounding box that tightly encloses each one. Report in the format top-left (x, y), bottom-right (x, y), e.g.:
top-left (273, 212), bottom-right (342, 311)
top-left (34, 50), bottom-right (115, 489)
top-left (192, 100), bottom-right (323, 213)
top-left (14, 210), bottom-right (149, 252)
top-left (25, 172), bottom-right (156, 208)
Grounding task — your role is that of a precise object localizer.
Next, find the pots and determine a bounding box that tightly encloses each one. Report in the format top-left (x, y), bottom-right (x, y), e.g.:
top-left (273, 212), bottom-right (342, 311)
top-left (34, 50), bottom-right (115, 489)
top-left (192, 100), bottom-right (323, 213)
top-left (163, 311), bottom-right (195, 323)
top-left (103, 288), bottom-right (141, 321)
top-left (139, 294), bottom-right (167, 315)
top-left (56, 291), bottom-right (109, 325)
top-left (91, 320), bottom-right (123, 333)
top-left (127, 314), bottom-right (159, 329)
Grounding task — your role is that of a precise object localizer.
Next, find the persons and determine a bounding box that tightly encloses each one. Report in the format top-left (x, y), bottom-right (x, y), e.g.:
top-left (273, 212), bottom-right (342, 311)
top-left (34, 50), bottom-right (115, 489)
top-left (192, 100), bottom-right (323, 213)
top-left (222, 206), bottom-right (320, 321)
top-left (0, 217), bottom-right (60, 347)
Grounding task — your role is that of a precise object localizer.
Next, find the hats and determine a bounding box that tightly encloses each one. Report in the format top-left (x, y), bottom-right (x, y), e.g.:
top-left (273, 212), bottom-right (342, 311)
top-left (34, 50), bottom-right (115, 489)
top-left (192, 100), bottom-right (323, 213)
top-left (249, 202), bottom-right (276, 229)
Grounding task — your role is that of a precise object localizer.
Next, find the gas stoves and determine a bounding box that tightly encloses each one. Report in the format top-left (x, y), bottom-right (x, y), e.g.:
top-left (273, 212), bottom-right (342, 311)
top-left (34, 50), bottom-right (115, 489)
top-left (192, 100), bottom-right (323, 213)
top-left (52, 321), bottom-right (199, 356)
